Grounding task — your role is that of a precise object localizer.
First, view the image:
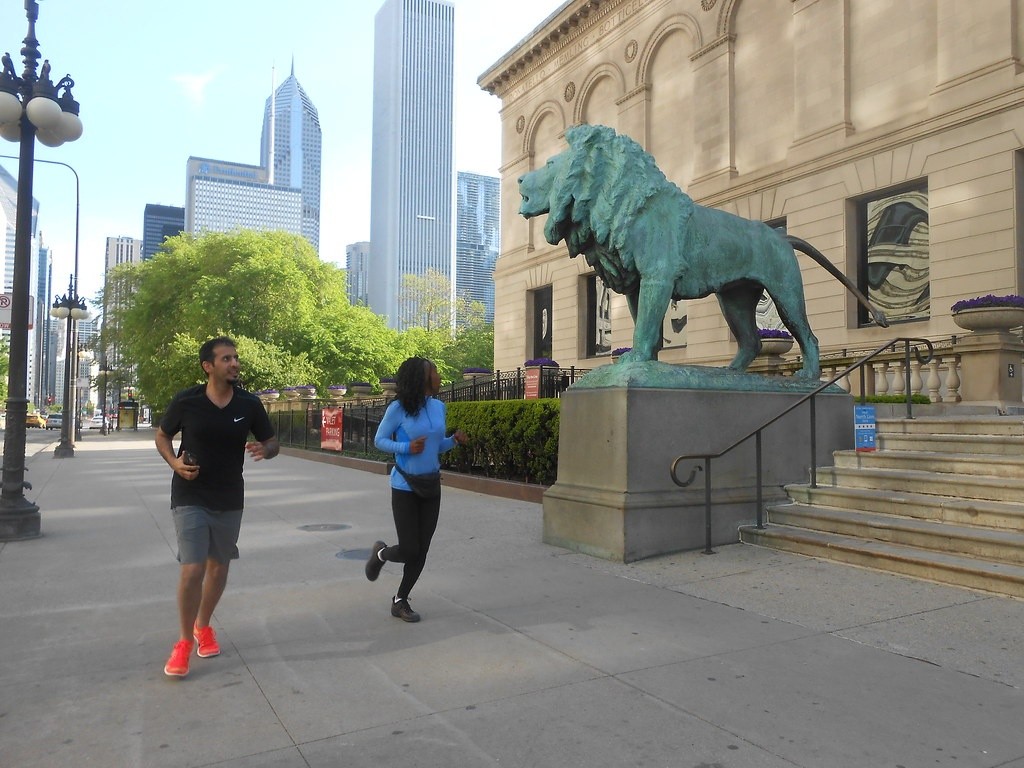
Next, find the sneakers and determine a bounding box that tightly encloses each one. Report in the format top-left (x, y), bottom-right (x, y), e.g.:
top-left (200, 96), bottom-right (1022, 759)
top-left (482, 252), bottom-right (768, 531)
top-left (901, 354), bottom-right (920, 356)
top-left (391, 595), bottom-right (420, 622)
top-left (164, 638), bottom-right (195, 677)
top-left (366, 540), bottom-right (388, 581)
top-left (192, 618), bottom-right (220, 658)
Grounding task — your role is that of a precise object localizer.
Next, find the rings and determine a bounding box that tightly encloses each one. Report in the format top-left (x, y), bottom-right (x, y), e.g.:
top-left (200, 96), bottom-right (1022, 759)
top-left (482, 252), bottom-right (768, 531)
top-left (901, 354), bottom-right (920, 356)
top-left (415, 444), bottom-right (418, 448)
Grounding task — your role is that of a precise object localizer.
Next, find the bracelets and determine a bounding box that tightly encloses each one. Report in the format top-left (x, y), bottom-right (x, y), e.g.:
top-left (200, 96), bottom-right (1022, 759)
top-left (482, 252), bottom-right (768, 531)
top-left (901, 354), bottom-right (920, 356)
top-left (452, 436), bottom-right (459, 444)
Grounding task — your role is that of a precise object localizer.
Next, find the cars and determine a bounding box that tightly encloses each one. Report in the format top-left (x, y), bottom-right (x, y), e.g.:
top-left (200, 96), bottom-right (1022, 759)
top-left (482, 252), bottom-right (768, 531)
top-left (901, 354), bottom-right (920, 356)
top-left (45, 413), bottom-right (63, 430)
top-left (88, 415), bottom-right (112, 429)
top-left (26, 414), bottom-right (46, 429)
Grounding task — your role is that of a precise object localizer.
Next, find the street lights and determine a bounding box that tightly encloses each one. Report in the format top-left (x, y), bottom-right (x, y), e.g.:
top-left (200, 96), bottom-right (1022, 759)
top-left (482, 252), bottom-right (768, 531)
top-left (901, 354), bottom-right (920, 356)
top-left (70, 331), bottom-right (94, 441)
top-left (0, 0), bottom-right (84, 545)
top-left (98, 355), bottom-right (114, 434)
top-left (49, 273), bottom-right (90, 460)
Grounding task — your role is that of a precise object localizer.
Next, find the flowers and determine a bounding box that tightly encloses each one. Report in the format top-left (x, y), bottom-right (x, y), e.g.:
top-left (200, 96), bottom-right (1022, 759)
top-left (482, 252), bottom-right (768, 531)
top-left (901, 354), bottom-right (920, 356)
top-left (612, 347), bottom-right (632, 355)
top-left (951, 294), bottom-right (1024, 312)
top-left (284, 387), bottom-right (296, 391)
top-left (254, 391), bottom-right (263, 396)
top-left (757, 329), bottom-right (793, 339)
top-left (327, 385), bottom-right (346, 389)
top-left (380, 378), bottom-right (396, 383)
top-left (524, 357), bottom-right (559, 368)
top-left (295, 384), bottom-right (314, 389)
top-left (264, 389), bottom-right (278, 394)
top-left (465, 368), bottom-right (491, 373)
top-left (350, 382), bottom-right (369, 387)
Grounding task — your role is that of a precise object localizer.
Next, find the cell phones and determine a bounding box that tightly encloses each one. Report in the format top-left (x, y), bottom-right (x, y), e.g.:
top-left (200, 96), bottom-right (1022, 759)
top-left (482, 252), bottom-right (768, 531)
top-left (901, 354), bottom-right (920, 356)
top-left (183, 452), bottom-right (198, 472)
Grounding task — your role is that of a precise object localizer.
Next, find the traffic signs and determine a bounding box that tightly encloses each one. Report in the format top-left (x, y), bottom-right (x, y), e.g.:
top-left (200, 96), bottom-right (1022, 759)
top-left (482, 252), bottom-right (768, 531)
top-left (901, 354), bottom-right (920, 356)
top-left (319, 406), bottom-right (343, 453)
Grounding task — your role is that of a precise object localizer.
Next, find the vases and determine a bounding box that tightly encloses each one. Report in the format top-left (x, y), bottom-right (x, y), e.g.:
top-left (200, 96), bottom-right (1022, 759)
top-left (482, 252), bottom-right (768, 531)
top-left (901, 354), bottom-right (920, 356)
top-left (351, 385), bottom-right (372, 397)
top-left (258, 395), bottom-right (268, 401)
top-left (296, 388), bottom-right (317, 398)
top-left (264, 393), bottom-right (280, 401)
top-left (759, 338), bottom-right (794, 356)
top-left (283, 391), bottom-right (301, 397)
top-left (381, 383), bottom-right (396, 396)
top-left (327, 389), bottom-right (348, 399)
top-left (611, 355), bottom-right (620, 364)
top-left (462, 373), bottom-right (491, 381)
top-left (527, 366), bottom-right (559, 376)
top-left (951, 306), bottom-right (1024, 331)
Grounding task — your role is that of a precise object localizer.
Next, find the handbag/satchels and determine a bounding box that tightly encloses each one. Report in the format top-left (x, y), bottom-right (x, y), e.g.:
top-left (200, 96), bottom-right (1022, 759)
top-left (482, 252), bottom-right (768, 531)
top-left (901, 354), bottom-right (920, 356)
top-left (395, 464), bottom-right (441, 500)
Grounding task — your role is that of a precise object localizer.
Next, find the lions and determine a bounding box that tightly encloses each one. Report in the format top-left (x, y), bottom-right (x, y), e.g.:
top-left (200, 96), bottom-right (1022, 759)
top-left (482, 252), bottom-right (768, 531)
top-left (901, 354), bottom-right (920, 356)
top-left (517, 124), bottom-right (889, 379)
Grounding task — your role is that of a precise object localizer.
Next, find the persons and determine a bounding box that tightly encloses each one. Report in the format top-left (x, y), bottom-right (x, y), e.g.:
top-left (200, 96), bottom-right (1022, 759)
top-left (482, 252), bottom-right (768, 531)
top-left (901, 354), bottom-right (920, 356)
top-left (365, 357), bottom-right (468, 622)
top-left (155, 338), bottom-right (279, 678)
